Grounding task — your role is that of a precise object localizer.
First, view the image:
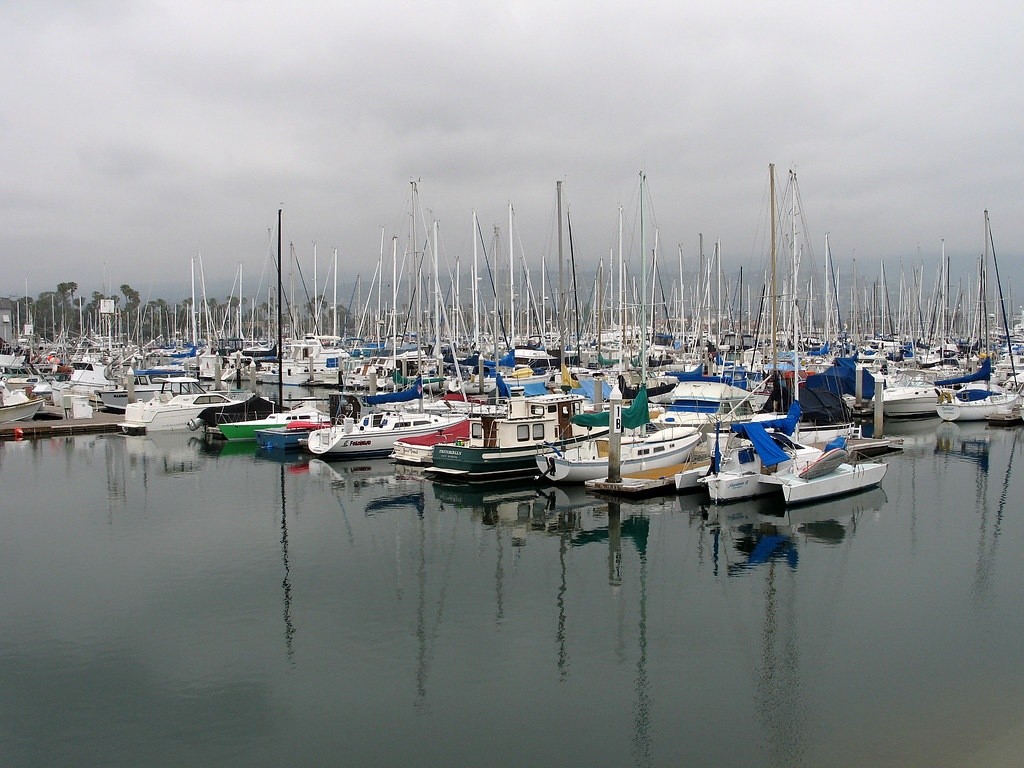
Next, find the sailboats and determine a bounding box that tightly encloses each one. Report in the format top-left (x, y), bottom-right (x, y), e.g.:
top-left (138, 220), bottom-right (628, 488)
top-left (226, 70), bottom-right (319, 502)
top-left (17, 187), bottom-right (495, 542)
top-left (0, 161), bottom-right (1024, 506)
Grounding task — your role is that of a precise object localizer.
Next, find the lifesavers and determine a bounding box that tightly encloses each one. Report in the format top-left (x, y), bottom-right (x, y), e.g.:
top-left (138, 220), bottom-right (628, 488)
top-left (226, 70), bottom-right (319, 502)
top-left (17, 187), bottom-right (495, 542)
top-left (31, 355), bottom-right (42, 365)
top-left (940, 392), bottom-right (951, 403)
